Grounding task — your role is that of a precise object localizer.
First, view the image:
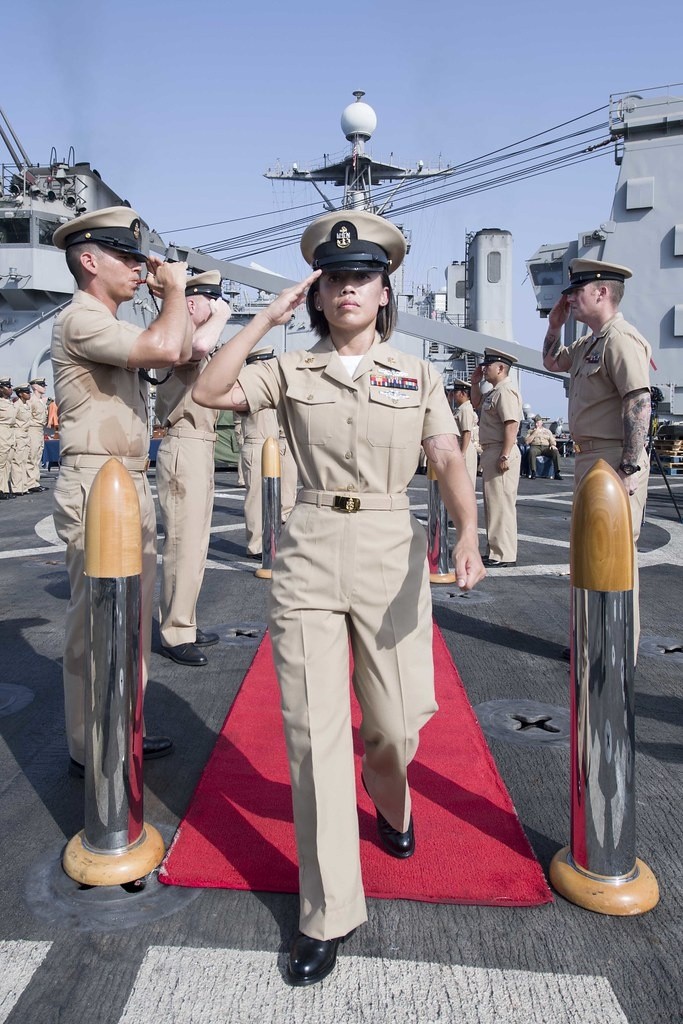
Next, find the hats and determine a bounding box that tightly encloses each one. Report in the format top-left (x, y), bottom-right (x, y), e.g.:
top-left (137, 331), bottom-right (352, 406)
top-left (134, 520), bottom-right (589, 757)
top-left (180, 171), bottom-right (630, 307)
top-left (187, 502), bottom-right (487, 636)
top-left (561, 257), bottom-right (633, 296)
top-left (535, 414), bottom-right (542, 423)
top-left (246, 345), bottom-right (275, 365)
top-left (301, 211), bottom-right (409, 276)
top-left (29, 377), bottom-right (46, 388)
top-left (451, 379), bottom-right (472, 392)
top-left (479, 346), bottom-right (518, 369)
top-left (0, 377), bottom-right (14, 388)
top-left (52, 207), bottom-right (149, 263)
top-left (12, 382), bottom-right (32, 394)
top-left (185, 269), bottom-right (230, 303)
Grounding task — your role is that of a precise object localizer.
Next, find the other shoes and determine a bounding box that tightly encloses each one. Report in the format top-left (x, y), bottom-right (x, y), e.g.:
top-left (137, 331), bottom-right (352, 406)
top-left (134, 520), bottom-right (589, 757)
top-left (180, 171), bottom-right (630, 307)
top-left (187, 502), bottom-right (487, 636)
top-left (561, 647), bottom-right (572, 662)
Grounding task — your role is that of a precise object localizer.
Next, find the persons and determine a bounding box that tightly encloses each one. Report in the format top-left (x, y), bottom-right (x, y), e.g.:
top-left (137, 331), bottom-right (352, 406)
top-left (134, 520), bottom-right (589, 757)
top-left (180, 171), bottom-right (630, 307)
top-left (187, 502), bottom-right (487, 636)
top-left (236, 342), bottom-right (298, 560)
top-left (524, 414), bottom-right (564, 480)
top-left (52, 206), bottom-right (193, 778)
top-left (468, 347), bottom-right (524, 569)
top-left (0, 377), bottom-right (58, 499)
top-left (193, 209), bottom-right (485, 988)
top-left (448, 376), bottom-right (478, 531)
top-left (154, 270), bottom-right (232, 668)
top-left (542, 258), bottom-right (652, 673)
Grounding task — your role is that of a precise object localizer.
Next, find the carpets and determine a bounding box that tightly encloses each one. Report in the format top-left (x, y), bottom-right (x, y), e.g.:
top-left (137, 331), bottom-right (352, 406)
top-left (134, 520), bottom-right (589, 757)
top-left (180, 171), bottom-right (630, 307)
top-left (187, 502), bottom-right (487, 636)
top-left (159, 609), bottom-right (555, 907)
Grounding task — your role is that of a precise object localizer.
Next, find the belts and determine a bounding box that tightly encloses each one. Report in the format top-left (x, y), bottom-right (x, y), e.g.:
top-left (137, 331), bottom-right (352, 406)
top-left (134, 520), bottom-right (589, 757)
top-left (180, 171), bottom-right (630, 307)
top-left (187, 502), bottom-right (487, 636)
top-left (165, 427), bottom-right (219, 443)
top-left (574, 439), bottom-right (629, 453)
top-left (61, 452), bottom-right (151, 472)
top-left (296, 488), bottom-right (410, 513)
top-left (1, 423), bottom-right (45, 432)
top-left (245, 438), bottom-right (268, 445)
top-left (481, 442), bottom-right (504, 450)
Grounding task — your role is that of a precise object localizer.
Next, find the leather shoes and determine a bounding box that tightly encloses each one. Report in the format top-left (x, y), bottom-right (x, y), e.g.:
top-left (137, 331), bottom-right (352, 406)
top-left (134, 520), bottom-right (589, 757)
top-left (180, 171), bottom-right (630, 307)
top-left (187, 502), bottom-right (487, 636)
top-left (247, 552), bottom-right (262, 560)
top-left (481, 555), bottom-right (516, 568)
top-left (287, 929), bottom-right (357, 984)
top-left (360, 773), bottom-right (415, 859)
top-left (159, 640), bottom-right (209, 665)
top-left (554, 474), bottom-right (563, 480)
top-left (143, 735), bottom-right (176, 759)
top-left (0, 485), bottom-right (49, 500)
top-left (194, 629), bottom-right (221, 647)
top-left (531, 473), bottom-right (536, 479)
top-left (69, 758), bottom-right (84, 777)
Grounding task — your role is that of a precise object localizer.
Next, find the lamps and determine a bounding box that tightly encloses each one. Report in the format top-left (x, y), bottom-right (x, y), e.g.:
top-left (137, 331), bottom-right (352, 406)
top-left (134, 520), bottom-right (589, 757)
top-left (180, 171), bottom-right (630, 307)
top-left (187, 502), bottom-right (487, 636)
top-left (10, 183), bottom-right (87, 213)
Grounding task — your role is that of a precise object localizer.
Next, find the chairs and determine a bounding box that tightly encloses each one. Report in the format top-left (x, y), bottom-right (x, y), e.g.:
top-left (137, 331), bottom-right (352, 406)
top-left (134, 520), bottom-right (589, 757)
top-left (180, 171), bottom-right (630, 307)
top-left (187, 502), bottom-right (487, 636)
top-left (535, 456), bottom-right (555, 479)
